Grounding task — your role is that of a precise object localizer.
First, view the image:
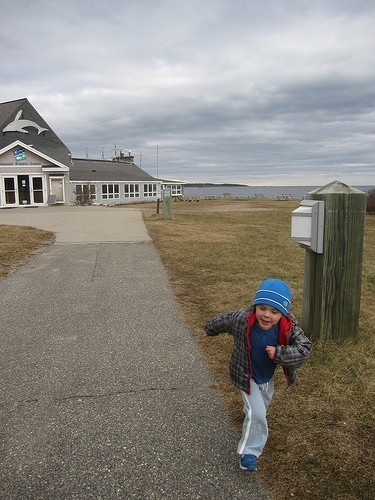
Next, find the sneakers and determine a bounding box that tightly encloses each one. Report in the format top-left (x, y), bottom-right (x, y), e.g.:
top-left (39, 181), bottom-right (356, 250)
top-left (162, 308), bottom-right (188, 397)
top-left (238, 453), bottom-right (259, 472)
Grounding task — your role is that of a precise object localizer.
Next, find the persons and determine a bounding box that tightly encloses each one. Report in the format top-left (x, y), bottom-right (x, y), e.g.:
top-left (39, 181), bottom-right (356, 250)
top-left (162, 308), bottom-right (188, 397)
top-left (202, 279), bottom-right (313, 471)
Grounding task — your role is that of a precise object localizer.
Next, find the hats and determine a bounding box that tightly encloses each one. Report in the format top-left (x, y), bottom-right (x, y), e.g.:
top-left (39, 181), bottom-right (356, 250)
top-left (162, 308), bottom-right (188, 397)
top-left (251, 279), bottom-right (292, 317)
